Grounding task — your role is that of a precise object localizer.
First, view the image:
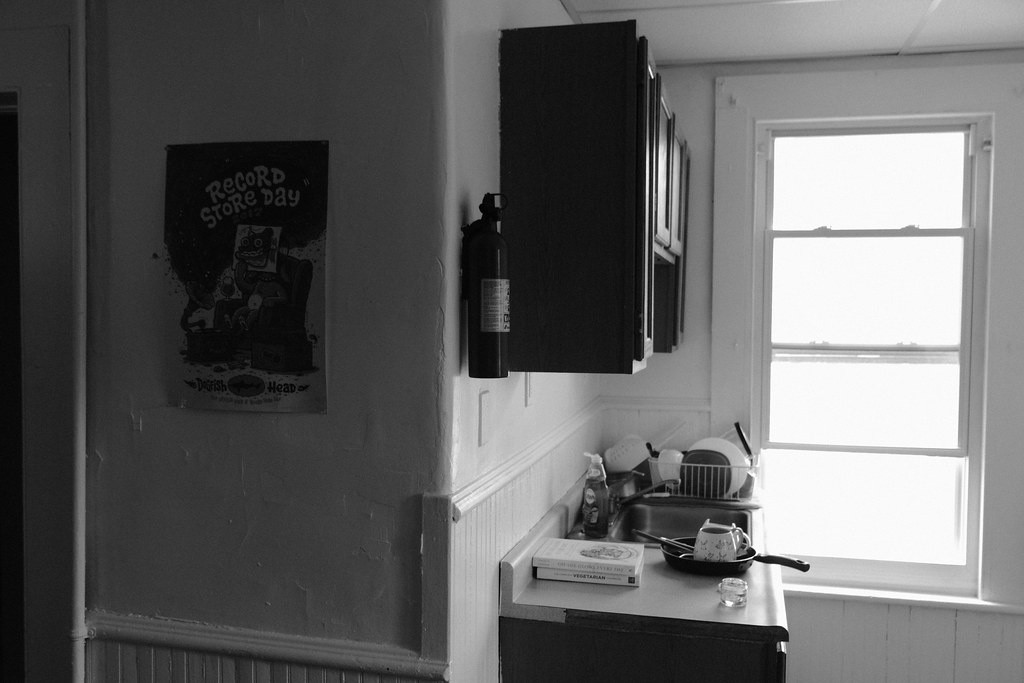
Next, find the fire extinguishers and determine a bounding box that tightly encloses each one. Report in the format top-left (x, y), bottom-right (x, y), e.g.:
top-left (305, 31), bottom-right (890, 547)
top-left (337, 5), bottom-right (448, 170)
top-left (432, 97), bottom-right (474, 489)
top-left (462, 190), bottom-right (512, 379)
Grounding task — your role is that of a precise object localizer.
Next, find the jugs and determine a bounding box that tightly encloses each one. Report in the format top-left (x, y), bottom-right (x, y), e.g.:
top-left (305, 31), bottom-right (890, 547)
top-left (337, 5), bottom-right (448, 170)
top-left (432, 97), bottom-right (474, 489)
top-left (701, 519), bottom-right (751, 555)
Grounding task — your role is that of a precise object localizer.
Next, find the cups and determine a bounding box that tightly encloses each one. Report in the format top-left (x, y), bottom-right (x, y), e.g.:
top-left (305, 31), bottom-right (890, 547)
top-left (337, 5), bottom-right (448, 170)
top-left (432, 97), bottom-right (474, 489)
top-left (694, 527), bottom-right (736, 562)
top-left (648, 456), bottom-right (665, 492)
top-left (720, 577), bottom-right (748, 608)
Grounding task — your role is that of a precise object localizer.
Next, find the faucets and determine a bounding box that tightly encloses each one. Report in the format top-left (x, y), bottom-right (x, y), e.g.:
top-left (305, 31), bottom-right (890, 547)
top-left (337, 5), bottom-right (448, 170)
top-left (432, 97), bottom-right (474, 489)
top-left (608, 472), bottom-right (679, 514)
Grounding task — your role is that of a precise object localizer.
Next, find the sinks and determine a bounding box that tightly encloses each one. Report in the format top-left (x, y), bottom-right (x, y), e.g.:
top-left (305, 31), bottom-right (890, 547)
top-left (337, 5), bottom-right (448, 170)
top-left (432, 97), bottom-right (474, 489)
top-left (609, 503), bottom-right (753, 549)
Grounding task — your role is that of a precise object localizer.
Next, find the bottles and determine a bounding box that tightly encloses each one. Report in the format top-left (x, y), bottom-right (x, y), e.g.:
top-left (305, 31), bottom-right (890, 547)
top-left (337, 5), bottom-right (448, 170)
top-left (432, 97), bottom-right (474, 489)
top-left (584, 453), bottom-right (609, 538)
top-left (681, 438), bottom-right (750, 499)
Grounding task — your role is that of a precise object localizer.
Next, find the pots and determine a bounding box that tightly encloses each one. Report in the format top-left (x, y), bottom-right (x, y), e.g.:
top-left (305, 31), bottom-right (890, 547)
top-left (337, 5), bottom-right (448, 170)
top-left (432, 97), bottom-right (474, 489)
top-left (661, 537), bottom-right (810, 575)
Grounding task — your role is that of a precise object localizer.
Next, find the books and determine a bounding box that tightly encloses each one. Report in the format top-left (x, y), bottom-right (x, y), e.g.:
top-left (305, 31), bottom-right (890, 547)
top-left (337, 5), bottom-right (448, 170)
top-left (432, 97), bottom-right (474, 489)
top-left (536, 564), bottom-right (645, 587)
top-left (532, 538), bottom-right (644, 575)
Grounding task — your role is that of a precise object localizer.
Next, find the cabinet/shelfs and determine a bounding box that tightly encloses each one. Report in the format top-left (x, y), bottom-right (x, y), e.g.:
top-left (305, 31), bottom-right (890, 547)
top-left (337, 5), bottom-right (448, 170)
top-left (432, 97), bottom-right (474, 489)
top-left (498, 618), bottom-right (787, 683)
top-left (498, 19), bottom-right (690, 376)
